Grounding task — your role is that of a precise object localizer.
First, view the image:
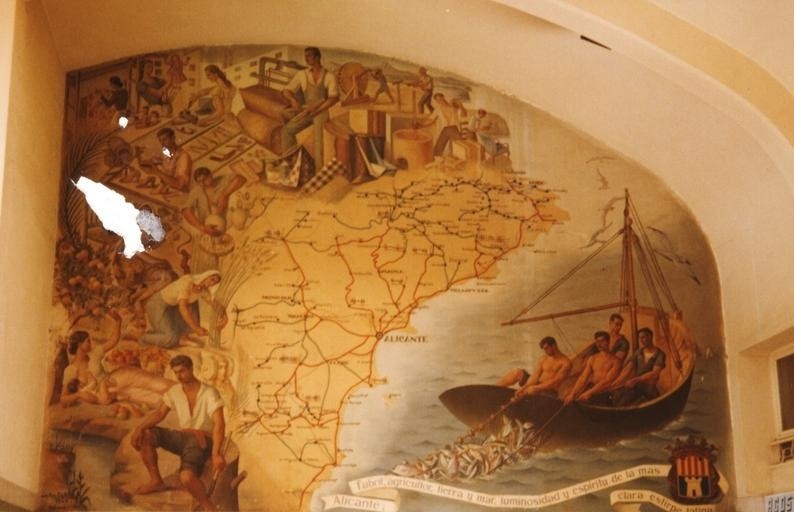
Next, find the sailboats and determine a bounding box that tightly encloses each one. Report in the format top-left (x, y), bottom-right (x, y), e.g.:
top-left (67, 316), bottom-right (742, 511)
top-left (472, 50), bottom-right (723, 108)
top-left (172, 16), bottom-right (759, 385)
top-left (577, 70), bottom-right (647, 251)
top-left (439, 188), bottom-right (698, 449)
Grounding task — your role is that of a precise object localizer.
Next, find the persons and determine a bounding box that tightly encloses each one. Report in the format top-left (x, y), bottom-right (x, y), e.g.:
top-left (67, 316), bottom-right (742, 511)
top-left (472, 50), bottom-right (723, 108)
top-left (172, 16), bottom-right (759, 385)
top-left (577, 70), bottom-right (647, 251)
top-left (562, 329), bottom-right (621, 408)
top-left (266, 44), bottom-right (513, 190)
top-left (576, 312), bottom-right (629, 369)
top-left (494, 334), bottom-right (573, 398)
top-left (609, 327), bottom-right (666, 408)
